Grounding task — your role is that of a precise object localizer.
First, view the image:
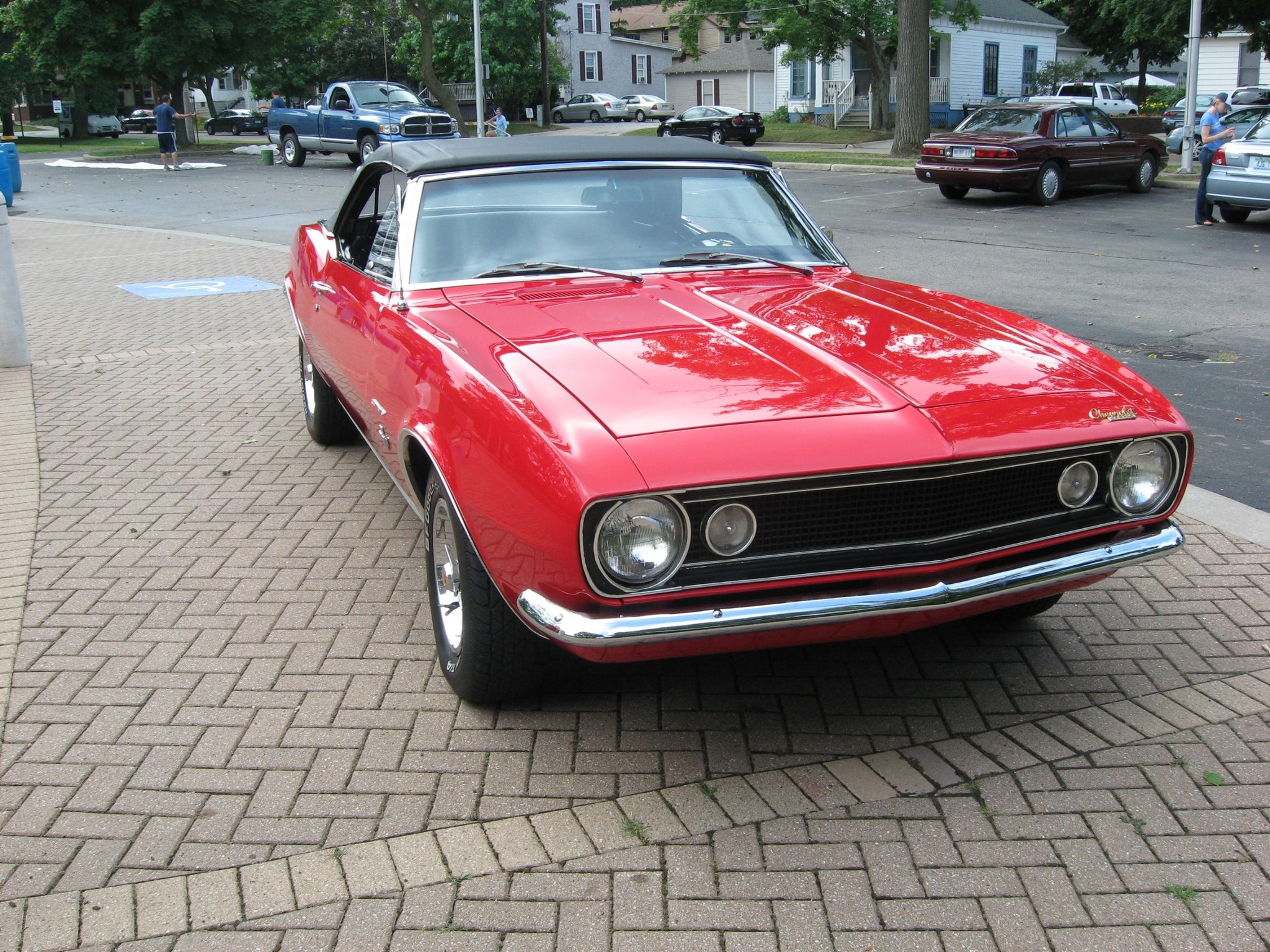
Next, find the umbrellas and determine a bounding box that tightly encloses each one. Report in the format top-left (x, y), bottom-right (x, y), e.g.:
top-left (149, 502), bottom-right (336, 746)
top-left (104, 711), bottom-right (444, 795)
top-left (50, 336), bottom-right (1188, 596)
top-left (1174, 70), bottom-right (1186, 88)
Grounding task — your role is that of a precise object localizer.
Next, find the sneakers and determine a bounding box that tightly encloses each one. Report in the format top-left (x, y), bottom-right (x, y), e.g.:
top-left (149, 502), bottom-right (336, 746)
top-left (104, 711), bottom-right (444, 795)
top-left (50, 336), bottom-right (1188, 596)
top-left (164, 166), bottom-right (173, 171)
top-left (174, 166), bottom-right (184, 170)
top-left (275, 158), bottom-right (286, 164)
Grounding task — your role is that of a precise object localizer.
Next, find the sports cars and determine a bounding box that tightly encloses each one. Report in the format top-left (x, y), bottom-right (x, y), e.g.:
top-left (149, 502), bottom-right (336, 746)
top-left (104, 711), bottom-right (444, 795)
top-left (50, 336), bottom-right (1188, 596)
top-left (286, 132), bottom-right (1197, 709)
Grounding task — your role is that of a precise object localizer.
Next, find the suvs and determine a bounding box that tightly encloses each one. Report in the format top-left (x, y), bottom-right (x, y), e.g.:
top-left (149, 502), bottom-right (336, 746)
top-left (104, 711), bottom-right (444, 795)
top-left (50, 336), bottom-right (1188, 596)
top-left (1224, 83), bottom-right (1270, 114)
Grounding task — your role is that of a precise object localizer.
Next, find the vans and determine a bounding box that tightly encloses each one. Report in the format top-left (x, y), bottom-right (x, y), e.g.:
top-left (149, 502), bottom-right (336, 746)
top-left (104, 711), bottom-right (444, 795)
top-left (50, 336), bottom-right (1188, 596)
top-left (58, 100), bottom-right (123, 139)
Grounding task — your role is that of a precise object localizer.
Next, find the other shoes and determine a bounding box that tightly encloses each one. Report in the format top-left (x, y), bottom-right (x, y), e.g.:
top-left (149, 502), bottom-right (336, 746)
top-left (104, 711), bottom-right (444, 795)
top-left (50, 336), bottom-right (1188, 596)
top-left (1197, 220), bottom-right (1212, 226)
top-left (1207, 217), bottom-right (1220, 223)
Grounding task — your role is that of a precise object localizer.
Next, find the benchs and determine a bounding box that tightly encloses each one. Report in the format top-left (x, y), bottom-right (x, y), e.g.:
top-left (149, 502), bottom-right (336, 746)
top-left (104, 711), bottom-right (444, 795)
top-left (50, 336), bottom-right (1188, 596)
top-left (367, 210), bottom-right (625, 285)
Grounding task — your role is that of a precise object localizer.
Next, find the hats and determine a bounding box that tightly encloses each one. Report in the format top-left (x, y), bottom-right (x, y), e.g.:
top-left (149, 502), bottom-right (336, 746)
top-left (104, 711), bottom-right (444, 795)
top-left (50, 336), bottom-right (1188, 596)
top-left (1216, 94), bottom-right (1232, 112)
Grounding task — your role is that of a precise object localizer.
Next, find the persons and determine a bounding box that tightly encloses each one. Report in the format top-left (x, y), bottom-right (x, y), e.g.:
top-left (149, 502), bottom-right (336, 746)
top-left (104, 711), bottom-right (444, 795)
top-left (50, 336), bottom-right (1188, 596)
top-left (486, 108), bottom-right (507, 137)
top-left (255, 87), bottom-right (286, 164)
top-left (142, 95), bottom-right (197, 170)
top-left (1195, 92), bottom-right (1236, 226)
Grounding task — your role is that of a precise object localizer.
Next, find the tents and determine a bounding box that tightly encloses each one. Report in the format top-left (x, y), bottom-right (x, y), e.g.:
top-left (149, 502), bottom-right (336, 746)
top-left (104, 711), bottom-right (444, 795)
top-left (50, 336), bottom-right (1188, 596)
top-left (1114, 73), bottom-right (1175, 100)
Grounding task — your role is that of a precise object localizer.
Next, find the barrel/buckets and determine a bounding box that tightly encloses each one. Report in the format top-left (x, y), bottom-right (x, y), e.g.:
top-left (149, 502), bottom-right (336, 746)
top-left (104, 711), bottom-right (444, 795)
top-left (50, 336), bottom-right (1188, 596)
top-left (261, 150), bottom-right (273, 165)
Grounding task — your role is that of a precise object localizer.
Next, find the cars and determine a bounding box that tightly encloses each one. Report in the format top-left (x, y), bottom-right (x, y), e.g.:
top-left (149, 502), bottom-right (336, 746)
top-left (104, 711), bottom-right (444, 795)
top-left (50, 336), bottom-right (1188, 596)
top-left (656, 105), bottom-right (765, 147)
top-left (118, 108), bottom-right (176, 135)
top-left (203, 108), bottom-right (268, 136)
top-left (550, 92), bottom-right (629, 123)
top-left (911, 102), bottom-right (1169, 206)
top-left (614, 94), bottom-right (675, 122)
top-left (1160, 94), bottom-right (1228, 135)
top-left (1205, 113), bottom-right (1270, 224)
top-left (1164, 104), bottom-right (1270, 160)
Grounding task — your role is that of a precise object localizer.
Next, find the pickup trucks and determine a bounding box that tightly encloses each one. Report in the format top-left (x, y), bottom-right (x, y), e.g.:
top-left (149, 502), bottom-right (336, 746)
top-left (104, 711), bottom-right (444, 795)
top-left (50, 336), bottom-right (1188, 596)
top-left (1003, 80), bottom-right (1139, 116)
top-left (263, 80), bottom-right (463, 167)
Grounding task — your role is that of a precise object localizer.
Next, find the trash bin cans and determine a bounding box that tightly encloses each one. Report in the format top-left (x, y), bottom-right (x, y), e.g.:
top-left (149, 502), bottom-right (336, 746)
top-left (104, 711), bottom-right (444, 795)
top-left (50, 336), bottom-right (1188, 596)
top-left (0, 143), bottom-right (22, 192)
top-left (0, 143), bottom-right (13, 206)
